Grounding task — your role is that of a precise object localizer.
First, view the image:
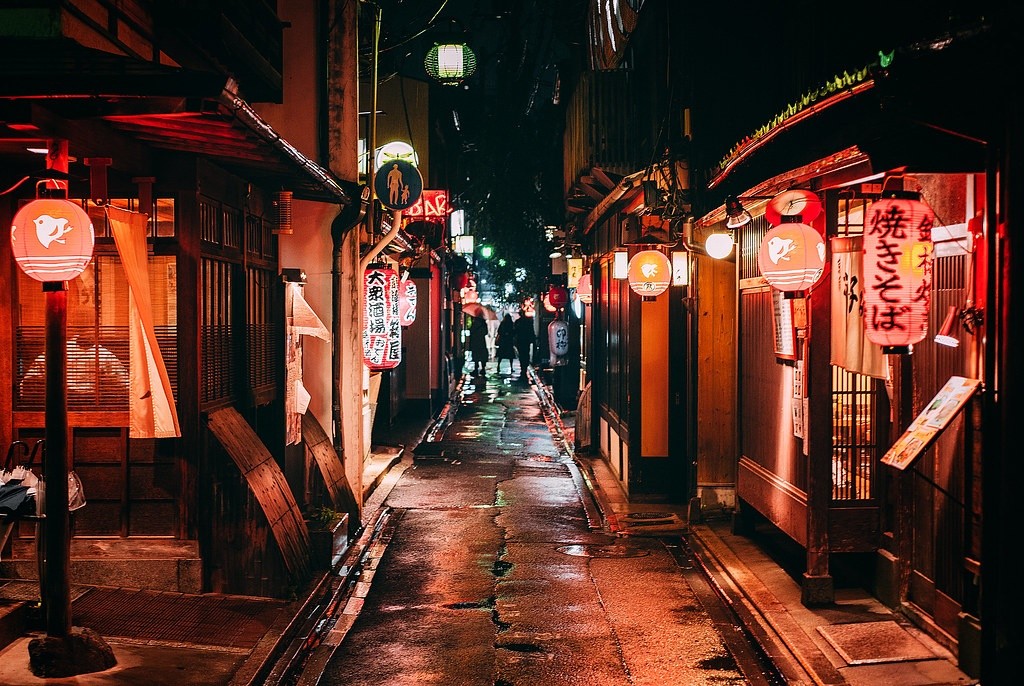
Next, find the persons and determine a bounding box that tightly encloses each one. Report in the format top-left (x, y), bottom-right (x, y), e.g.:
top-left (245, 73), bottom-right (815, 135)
top-left (494, 313), bottom-right (514, 375)
top-left (513, 309), bottom-right (534, 379)
top-left (469, 307), bottom-right (490, 376)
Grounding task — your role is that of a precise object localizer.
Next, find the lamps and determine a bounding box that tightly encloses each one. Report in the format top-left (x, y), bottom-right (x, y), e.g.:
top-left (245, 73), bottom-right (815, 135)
top-left (725, 194), bottom-right (775, 229)
top-left (936, 304), bottom-right (966, 346)
top-left (280, 268), bottom-right (308, 284)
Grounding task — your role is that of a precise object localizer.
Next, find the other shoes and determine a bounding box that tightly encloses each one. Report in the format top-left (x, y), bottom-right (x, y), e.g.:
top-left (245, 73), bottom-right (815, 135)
top-left (494, 371), bottom-right (499, 375)
top-left (511, 368), bottom-right (515, 372)
top-left (480, 369), bottom-right (485, 373)
top-left (470, 370), bottom-right (478, 375)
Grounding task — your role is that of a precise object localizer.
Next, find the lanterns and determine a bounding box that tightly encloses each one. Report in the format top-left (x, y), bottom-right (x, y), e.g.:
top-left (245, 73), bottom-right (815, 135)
top-left (451, 267), bottom-right (480, 306)
top-left (359, 260), bottom-right (401, 372)
top-left (399, 275), bottom-right (417, 330)
top-left (626, 245), bottom-right (674, 302)
top-left (850, 189), bottom-right (933, 355)
top-left (8, 189), bottom-right (97, 293)
top-left (545, 272), bottom-right (593, 358)
top-left (755, 215), bottom-right (828, 299)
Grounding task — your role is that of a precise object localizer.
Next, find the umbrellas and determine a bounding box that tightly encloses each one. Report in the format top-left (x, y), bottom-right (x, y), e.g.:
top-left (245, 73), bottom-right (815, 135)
top-left (461, 302), bottom-right (498, 321)
